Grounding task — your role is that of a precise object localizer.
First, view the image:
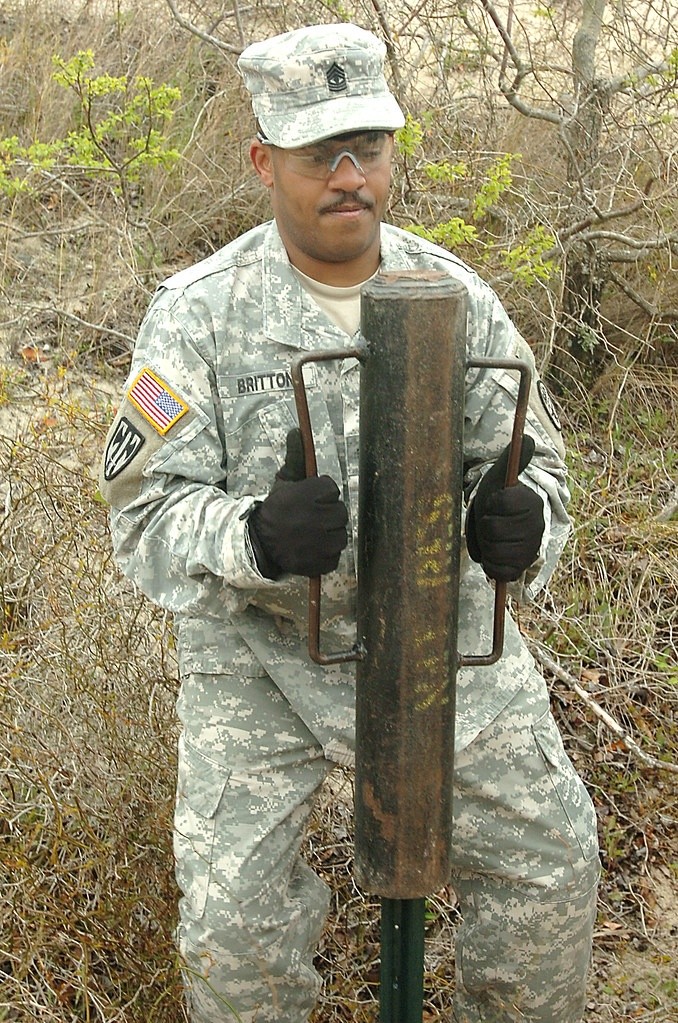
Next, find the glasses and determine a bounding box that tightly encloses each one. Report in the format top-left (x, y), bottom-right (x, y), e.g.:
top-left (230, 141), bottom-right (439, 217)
top-left (269, 129), bottom-right (394, 179)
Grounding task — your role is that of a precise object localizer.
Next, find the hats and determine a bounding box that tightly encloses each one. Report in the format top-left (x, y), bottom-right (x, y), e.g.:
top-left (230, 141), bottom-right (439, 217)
top-left (237, 23), bottom-right (406, 150)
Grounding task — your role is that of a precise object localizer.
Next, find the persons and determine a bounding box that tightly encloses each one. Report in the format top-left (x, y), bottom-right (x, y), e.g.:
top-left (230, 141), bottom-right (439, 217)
top-left (101, 24), bottom-right (601, 1023)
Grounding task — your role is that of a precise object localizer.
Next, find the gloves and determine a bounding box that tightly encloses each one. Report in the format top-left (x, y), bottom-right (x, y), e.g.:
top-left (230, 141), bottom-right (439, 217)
top-left (464, 434), bottom-right (546, 582)
top-left (237, 429), bottom-right (348, 576)
